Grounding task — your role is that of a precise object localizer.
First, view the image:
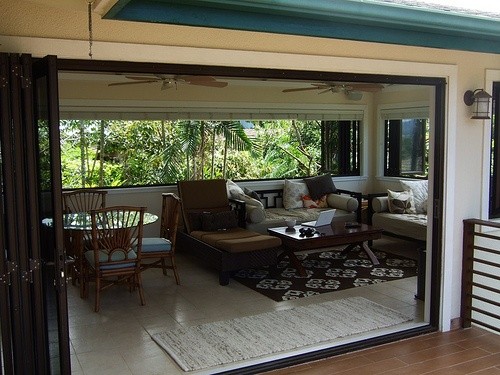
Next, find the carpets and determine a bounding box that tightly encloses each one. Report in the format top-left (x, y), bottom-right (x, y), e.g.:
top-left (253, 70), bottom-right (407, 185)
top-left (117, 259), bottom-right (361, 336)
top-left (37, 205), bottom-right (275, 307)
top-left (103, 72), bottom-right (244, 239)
top-left (227, 247), bottom-right (417, 301)
top-left (152, 296), bottom-right (413, 371)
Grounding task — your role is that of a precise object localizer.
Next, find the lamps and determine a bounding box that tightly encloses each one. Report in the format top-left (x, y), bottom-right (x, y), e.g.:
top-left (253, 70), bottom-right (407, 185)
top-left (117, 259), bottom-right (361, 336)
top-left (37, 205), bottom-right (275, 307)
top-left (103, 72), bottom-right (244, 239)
top-left (464, 88), bottom-right (494, 119)
top-left (347, 91), bottom-right (362, 100)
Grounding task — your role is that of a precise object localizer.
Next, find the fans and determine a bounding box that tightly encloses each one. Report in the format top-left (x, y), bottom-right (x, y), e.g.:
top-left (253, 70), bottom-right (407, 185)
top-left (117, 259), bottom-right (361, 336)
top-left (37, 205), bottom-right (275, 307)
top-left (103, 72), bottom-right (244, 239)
top-left (107, 74), bottom-right (229, 91)
top-left (282, 81), bottom-right (385, 96)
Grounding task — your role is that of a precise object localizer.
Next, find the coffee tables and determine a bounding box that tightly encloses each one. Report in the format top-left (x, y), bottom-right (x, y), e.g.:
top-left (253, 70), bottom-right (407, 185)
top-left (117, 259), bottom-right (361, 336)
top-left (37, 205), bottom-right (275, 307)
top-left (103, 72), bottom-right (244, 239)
top-left (267, 221), bottom-right (385, 277)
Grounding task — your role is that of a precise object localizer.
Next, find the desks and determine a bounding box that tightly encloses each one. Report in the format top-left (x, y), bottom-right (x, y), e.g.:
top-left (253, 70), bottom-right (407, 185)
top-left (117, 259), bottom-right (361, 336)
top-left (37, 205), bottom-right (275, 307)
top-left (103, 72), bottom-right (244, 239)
top-left (41, 211), bottom-right (159, 298)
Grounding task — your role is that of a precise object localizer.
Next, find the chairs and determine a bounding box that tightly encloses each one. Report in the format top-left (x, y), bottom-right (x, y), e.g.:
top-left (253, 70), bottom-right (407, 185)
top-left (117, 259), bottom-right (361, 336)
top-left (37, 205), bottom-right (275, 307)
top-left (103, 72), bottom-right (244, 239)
top-left (65, 189), bottom-right (181, 312)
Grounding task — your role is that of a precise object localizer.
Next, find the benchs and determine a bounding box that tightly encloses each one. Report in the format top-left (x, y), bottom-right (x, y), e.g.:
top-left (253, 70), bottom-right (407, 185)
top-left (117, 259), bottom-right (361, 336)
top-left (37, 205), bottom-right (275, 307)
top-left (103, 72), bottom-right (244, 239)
top-left (368, 190), bottom-right (427, 247)
top-left (174, 177), bottom-right (282, 286)
top-left (229, 189), bottom-right (362, 234)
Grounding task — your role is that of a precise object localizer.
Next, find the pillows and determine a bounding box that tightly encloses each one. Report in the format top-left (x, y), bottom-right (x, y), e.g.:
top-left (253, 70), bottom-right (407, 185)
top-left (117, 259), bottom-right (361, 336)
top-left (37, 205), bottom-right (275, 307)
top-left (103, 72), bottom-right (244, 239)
top-left (283, 172), bottom-right (336, 209)
top-left (327, 194), bottom-right (358, 212)
top-left (225, 179), bottom-right (266, 223)
top-left (387, 189), bottom-right (417, 214)
top-left (372, 195), bottom-right (388, 213)
top-left (400, 180), bottom-right (428, 213)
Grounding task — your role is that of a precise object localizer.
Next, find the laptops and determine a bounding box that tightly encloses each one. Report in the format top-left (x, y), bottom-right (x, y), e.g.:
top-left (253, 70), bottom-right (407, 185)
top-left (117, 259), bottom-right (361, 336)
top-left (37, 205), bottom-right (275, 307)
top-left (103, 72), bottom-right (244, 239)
top-left (301, 209), bottom-right (336, 227)
